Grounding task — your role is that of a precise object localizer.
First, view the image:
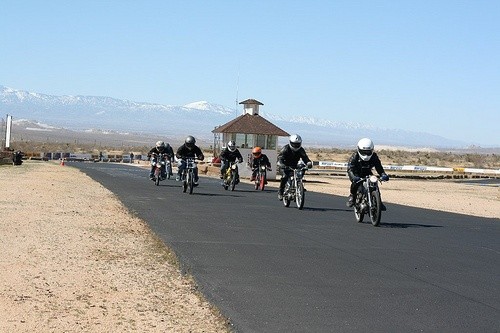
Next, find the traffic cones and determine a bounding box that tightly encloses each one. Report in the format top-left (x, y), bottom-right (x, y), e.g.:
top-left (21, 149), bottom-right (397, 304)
top-left (60, 159), bottom-right (64, 166)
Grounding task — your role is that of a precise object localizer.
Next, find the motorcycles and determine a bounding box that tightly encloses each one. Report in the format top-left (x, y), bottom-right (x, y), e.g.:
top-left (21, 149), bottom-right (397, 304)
top-left (281, 161), bottom-right (311, 210)
top-left (180, 156), bottom-right (201, 194)
top-left (163, 155), bottom-right (173, 180)
top-left (352, 174), bottom-right (388, 226)
top-left (222, 158), bottom-right (241, 191)
top-left (150, 152), bottom-right (170, 187)
top-left (249, 162), bottom-right (272, 191)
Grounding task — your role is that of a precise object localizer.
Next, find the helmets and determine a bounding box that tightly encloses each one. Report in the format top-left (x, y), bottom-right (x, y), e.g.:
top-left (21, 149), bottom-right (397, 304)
top-left (164, 143), bottom-right (170, 151)
top-left (228, 140), bottom-right (237, 152)
top-left (185, 136), bottom-right (195, 149)
top-left (356, 138), bottom-right (374, 161)
top-left (252, 147), bottom-right (261, 158)
top-left (289, 134), bottom-right (302, 152)
top-left (156, 141), bottom-right (164, 152)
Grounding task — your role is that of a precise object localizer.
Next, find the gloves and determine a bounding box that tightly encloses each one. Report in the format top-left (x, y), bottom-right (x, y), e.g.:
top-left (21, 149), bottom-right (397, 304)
top-left (353, 177), bottom-right (360, 183)
top-left (382, 175), bottom-right (389, 181)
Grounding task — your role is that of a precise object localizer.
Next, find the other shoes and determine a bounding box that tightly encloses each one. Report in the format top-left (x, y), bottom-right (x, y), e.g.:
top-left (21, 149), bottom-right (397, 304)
top-left (194, 182), bottom-right (199, 184)
top-left (176, 175), bottom-right (181, 182)
top-left (346, 195), bottom-right (354, 207)
top-left (381, 202), bottom-right (386, 211)
top-left (278, 193), bottom-right (283, 200)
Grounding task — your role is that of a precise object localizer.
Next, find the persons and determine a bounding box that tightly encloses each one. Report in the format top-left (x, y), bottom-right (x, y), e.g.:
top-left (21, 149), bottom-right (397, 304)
top-left (248, 147), bottom-right (272, 185)
top-left (276, 134), bottom-right (312, 201)
top-left (219, 140), bottom-right (243, 185)
top-left (147, 141), bottom-right (171, 181)
top-left (11, 150), bottom-right (24, 166)
top-left (130, 152), bottom-right (134, 164)
top-left (99, 152), bottom-right (104, 160)
top-left (176, 136), bottom-right (204, 187)
top-left (345, 138), bottom-right (389, 211)
top-left (164, 143), bottom-right (174, 176)
